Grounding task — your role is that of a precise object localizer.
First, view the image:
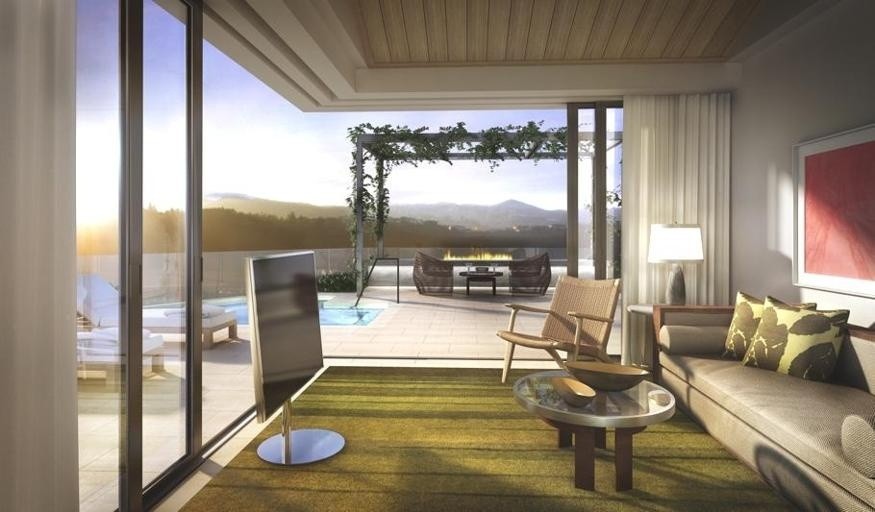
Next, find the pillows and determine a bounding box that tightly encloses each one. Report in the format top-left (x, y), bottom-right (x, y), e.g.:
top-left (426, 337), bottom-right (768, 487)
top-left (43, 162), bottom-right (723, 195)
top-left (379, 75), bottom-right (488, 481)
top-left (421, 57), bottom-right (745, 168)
top-left (720, 289), bottom-right (849, 384)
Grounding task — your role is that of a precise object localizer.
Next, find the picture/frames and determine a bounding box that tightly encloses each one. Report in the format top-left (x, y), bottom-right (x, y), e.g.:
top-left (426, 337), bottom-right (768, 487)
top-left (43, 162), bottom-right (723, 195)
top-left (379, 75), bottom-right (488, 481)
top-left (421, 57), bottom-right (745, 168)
top-left (791, 123), bottom-right (875, 301)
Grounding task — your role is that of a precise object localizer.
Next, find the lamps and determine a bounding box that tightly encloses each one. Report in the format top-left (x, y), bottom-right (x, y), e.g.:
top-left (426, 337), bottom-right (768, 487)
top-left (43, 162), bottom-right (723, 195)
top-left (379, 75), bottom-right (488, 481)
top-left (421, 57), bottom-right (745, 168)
top-left (646, 221), bottom-right (705, 306)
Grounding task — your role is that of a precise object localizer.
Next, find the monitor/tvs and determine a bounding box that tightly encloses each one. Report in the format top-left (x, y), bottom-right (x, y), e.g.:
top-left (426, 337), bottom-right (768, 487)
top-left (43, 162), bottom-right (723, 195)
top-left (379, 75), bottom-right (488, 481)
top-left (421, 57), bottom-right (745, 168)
top-left (244, 249), bottom-right (324, 424)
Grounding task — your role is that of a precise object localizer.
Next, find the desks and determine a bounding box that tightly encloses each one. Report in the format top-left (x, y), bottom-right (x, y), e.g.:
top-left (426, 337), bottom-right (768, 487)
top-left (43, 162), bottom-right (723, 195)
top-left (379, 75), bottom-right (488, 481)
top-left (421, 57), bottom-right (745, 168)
top-left (627, 305), bottom-right (653, 371)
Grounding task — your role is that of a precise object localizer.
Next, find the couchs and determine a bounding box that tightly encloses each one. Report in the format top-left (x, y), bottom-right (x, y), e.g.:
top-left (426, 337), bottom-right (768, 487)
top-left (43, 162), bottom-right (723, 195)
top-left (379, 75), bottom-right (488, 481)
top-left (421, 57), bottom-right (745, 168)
top-left (653, 302), bottom-right (875, 512)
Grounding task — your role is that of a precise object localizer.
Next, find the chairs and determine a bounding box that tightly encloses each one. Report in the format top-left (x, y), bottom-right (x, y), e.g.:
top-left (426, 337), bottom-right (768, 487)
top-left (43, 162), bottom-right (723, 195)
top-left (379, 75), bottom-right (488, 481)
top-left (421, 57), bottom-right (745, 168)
top-left (508, 252), bottom-right (551, 298)
top-left (413, 249), bottom-right (454, 295)
top-left (496, 272), bottom-right (621, 384)
top-left (76, 272), bottom-right (237, 392)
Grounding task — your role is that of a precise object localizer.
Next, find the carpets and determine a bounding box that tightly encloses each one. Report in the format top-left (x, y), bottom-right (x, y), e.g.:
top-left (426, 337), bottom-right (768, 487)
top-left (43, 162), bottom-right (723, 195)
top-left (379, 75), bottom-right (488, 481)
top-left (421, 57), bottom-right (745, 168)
top-left (177, 366), bottom-right (803, 512)
